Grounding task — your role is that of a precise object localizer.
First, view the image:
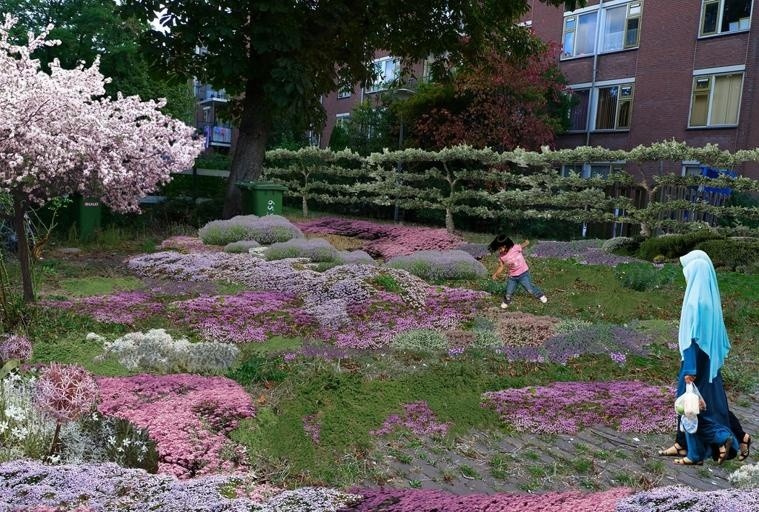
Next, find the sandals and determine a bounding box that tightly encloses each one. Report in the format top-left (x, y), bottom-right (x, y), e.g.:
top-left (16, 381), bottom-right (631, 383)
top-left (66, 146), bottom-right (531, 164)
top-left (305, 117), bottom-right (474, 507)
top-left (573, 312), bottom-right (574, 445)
top-left (671, 457), bottom-right (704, 465)
top-left (658, 442), bottom-right (687, 457)
top-left (738, 431), bottom-right (751, 462)
top-left (717, 435), bottom-right (733, 465)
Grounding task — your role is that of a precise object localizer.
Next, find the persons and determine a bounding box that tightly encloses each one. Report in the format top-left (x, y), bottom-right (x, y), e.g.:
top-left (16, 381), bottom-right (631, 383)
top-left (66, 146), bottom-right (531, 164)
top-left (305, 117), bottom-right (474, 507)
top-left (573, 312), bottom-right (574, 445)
top-left (486, 234), bottom-right (547, 309)
top-left (659, 250), bottom-right (752, 460)
top-left (670, 257), bottom-right (735, 466)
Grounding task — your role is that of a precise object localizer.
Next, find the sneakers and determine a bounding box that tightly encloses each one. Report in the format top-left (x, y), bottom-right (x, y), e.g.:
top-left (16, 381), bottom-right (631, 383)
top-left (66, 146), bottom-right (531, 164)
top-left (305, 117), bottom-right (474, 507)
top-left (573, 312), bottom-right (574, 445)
top-left (500, 300), bottom-right (512, 309)
top-left (539, 294), bottom-right (548, 304)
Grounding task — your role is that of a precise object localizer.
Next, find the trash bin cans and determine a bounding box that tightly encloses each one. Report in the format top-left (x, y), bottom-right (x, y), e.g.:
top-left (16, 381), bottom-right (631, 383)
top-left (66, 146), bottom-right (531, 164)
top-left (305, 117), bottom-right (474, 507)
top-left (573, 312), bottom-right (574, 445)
top-left (233, 180), bottom-right (288, 217)
top-left (63, 196), bottom-right (103, 241)
